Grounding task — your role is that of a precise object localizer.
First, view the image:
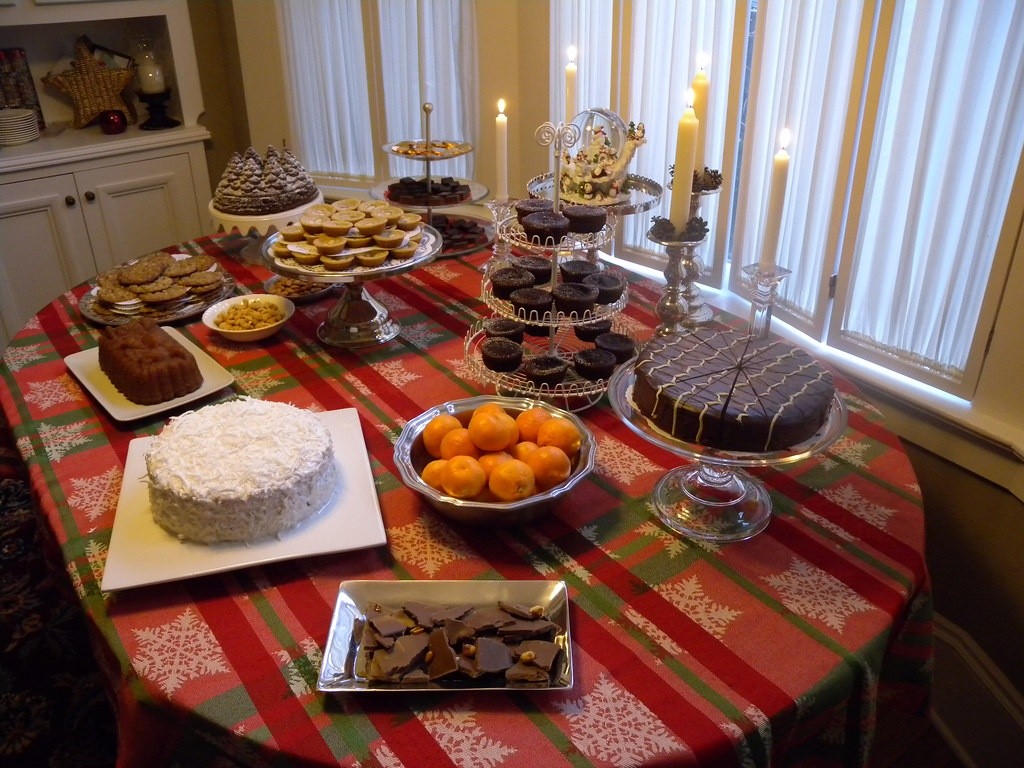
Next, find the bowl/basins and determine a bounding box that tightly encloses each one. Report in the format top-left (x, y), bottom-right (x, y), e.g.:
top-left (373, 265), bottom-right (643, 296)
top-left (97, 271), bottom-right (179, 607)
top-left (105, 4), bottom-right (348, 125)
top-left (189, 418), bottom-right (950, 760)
top-left (388, 393), bottom-right (596, 525)
top-left (201, 293), bottom-right (296, 342)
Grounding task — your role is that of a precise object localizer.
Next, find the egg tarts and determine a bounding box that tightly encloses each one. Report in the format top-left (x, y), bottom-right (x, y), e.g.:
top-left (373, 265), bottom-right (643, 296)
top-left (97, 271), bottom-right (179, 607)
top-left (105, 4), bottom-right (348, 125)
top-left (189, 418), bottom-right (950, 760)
top-left (272, 198), bottom-right (422, 268)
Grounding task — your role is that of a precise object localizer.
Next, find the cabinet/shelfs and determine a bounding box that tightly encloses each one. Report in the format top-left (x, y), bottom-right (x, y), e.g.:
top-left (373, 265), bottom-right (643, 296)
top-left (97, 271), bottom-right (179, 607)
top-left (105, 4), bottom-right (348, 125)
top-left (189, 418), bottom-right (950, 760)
top-left (1, 0), bottom-right (215, 360)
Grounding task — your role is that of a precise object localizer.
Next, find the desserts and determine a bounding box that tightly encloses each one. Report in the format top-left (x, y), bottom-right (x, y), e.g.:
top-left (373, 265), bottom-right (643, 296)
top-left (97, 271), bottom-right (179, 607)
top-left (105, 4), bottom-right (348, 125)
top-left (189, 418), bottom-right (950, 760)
top-left (385, 139), bottom-right (487, 254)
top-left (480, 198), bottom-right (635, 388)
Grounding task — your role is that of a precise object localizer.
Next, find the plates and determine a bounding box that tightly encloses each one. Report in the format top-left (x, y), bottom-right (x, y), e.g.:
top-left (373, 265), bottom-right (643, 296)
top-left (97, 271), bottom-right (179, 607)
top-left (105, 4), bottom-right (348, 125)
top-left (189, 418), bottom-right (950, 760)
top-left (316, 580), bottom-right (575, 693)
top-left (417, 212), bottom-right (500, 258)
top-left (0, 108), bottom-right (40, 146)
top-left (76, 267), bottom-right (235, 327)
top-left (61, 323), bottom-right (238, 424)
top-left (378, 137), bottom-right (473, 161)
top-left (367, 176), bottom-right (488, 206)
top-left (100, 406), bottom-right (388, 592)
top-left (524, 169), bottom-right (664, 272)
top-left (262, 270), bottom-right (337, 304)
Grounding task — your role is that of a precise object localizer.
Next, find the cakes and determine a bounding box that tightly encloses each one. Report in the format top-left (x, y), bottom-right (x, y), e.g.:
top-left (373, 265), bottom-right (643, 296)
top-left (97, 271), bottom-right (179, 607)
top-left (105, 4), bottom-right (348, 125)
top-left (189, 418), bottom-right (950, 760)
top-left (214, 145), bottom-right (320, 217)
top-left (633, 327), bottom-right (835, 452)
top-left (137, 396), bottom-right (336, 545)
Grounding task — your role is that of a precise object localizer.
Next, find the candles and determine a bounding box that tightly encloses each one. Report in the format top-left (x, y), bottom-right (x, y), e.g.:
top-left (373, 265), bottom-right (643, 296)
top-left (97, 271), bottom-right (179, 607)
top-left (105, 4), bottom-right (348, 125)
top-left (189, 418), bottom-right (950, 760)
top-left (757, 127), bottom-right (792, 277)
top-left (565, 45), bottom-right (577, 125)
top-left (496, 98), bottom-right (508, 205)
top-left (667, 87), bottom-right (699, 240)
top-left (690, 51), bottom-right (710, 181)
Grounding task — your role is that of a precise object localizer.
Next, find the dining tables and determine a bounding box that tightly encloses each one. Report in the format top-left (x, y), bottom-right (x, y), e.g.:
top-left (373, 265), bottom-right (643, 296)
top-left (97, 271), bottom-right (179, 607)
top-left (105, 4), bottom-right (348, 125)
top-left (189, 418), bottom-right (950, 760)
top-left (0, 223), bottom-right (928, 768)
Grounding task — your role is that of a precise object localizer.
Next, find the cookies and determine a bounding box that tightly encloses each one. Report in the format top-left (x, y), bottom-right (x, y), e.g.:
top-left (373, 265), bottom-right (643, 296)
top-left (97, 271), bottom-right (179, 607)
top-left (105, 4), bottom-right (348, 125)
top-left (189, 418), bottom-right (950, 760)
top-left (91, 251), bottom-right (224, 322)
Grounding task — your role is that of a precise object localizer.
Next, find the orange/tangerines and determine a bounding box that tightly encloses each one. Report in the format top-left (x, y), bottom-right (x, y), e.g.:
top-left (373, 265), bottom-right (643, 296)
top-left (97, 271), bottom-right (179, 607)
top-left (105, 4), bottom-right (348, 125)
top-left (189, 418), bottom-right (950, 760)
top-left (422, 403), bottom-right (581, 503)
